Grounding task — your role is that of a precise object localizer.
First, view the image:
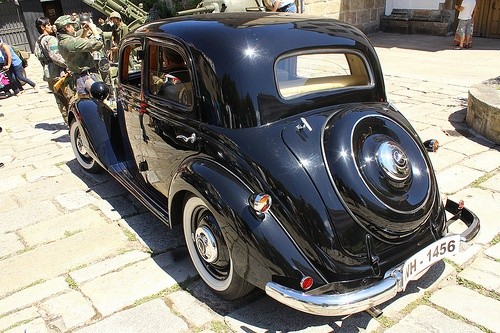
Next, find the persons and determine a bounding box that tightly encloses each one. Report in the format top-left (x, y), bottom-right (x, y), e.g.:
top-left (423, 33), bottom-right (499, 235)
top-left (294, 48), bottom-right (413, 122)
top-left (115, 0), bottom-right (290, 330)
top-left (263, 0), bottom-right (298, 13)
top-left (162, 48), bottom-right (185, 69)
top-left (454, 0), bottom-right (475, 51)
top-left (0, 12), bottom-right (127, 123)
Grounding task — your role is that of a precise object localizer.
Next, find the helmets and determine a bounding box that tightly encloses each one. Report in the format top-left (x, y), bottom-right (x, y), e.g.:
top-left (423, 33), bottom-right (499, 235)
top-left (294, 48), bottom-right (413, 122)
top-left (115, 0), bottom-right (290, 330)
top-left (109, 11), bottom-right (121, 19)
top-left (79, 15), bottom-right (90, 25)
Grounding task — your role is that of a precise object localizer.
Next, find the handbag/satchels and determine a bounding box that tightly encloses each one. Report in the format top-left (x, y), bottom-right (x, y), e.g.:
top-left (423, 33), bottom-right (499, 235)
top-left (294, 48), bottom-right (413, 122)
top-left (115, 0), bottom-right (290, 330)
top-left (43, 62), bottom-right (63, 80)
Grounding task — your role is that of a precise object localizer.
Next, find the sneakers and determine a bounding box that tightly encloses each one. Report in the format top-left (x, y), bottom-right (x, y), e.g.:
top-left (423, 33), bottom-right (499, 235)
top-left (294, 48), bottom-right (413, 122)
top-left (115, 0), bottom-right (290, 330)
top-left (453, 45), bottom-right (470, 50)
top-left (13, 85), bottom-right (40, 94)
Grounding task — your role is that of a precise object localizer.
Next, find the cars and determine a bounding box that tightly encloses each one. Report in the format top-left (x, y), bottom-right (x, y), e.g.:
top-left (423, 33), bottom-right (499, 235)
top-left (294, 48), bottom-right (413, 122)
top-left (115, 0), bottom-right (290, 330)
top-left (65, 11), bottom-right (481, 319)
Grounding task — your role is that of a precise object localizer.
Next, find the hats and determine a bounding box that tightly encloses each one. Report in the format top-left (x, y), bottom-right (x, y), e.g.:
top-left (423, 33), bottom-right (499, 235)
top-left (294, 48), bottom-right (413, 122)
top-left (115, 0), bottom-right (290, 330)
top-left (54, 15), bottom-right (77, 31)
